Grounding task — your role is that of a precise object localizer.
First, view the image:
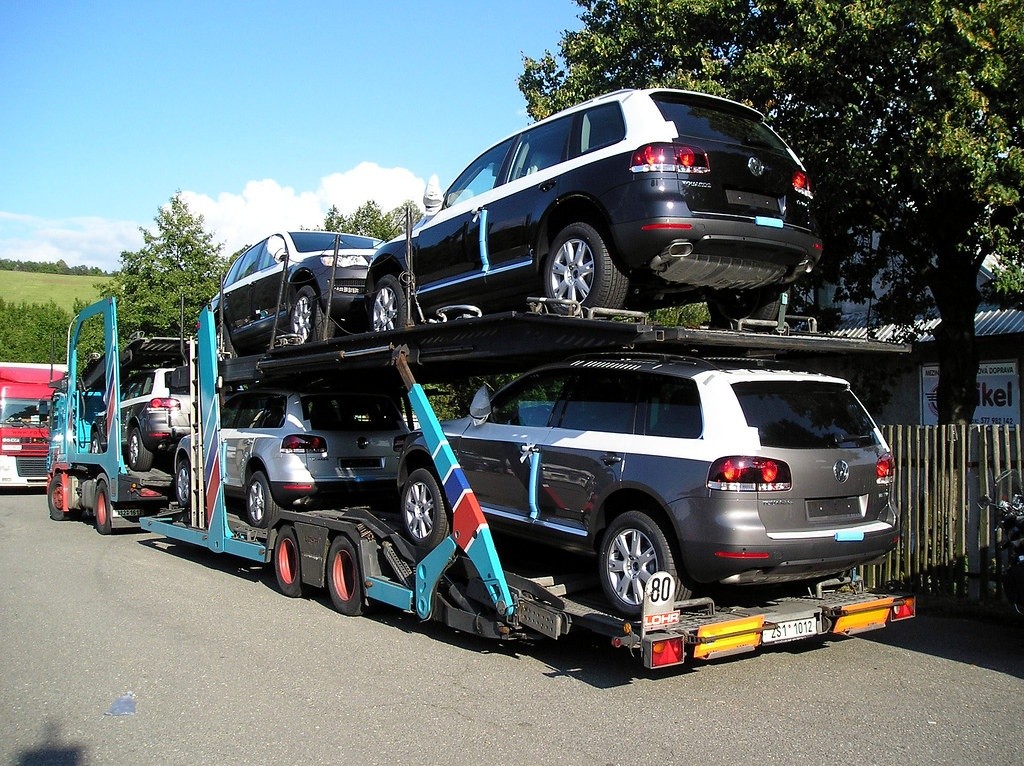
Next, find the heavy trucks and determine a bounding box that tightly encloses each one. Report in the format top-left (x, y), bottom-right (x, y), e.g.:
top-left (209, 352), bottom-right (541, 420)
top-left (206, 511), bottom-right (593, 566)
top-left (0, 362), bottom-right (68, 488)
top-left (37, 294), bottom-right (918, 672)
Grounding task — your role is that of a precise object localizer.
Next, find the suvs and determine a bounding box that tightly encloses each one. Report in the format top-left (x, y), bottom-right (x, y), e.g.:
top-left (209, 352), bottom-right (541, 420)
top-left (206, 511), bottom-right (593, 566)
top-left (173, 384), bottom-right (413, 530)
top-left (364, 87), bottom-right (823, 336)
top-left (198, 231), bottom-right (385, 354)
top-left (93, 365), bottom-right (196, 469)
top-left (397, 353), bottom-right (900, 613)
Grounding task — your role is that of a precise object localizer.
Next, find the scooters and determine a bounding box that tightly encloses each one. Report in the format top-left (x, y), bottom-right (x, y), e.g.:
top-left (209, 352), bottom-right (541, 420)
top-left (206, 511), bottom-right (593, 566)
top-left (976, 470), bottom-right (1024, 617)
